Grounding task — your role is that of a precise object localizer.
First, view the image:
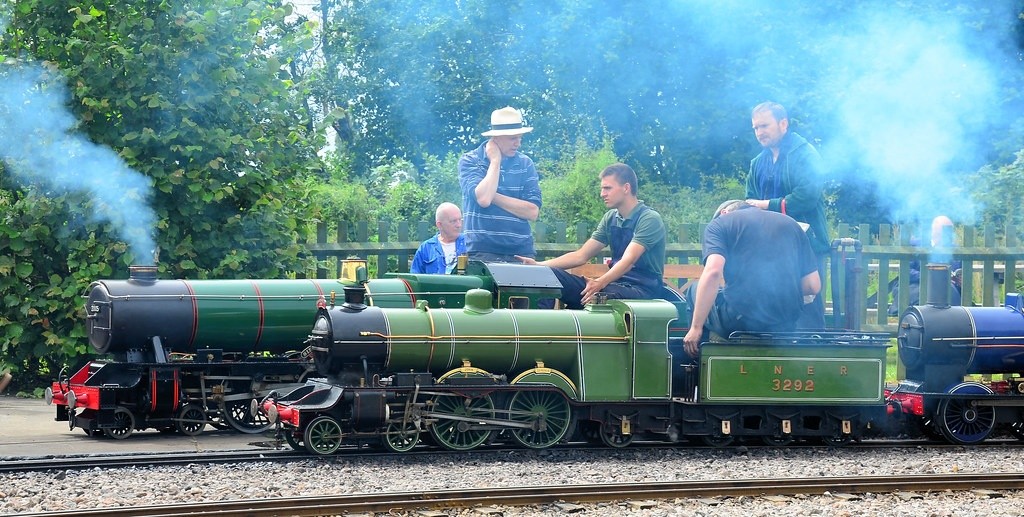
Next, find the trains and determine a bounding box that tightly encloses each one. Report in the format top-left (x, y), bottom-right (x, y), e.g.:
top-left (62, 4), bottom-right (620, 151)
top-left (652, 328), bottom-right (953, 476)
top-left (44, 252), bottom-right (1024, 455)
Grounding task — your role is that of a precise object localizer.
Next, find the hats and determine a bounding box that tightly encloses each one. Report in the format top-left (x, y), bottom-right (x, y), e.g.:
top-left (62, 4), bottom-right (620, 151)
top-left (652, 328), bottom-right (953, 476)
top-left (481, 107), bottom-right (533, 137)
top-left (713, 200), bottom-right (742, 219)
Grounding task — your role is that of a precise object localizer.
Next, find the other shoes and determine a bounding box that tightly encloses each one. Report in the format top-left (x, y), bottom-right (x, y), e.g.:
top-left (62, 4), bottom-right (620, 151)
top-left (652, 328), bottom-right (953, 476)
top-left (673, 377), bottom-right (699, 398)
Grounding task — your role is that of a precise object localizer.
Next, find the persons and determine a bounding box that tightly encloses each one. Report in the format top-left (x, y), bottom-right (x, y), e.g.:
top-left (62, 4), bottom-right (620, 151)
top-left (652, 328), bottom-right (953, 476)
top-left (514, 163), bottom-right (665, 310)
top-left (745, 101), bottom-right (832, 331)
top-left (458, 107), bottom-right (542, 263)
top-left (889, 216), bottom-right (962, 317)
top-left (410, 202), bottom-right (466, 274)
top-left (672, 201), bottom-right (821, 402)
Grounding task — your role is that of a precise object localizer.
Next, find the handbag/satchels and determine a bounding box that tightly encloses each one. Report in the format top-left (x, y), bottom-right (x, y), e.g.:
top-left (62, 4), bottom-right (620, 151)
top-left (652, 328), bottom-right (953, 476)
top-left (781, 198), bottom-right (820, 306)
